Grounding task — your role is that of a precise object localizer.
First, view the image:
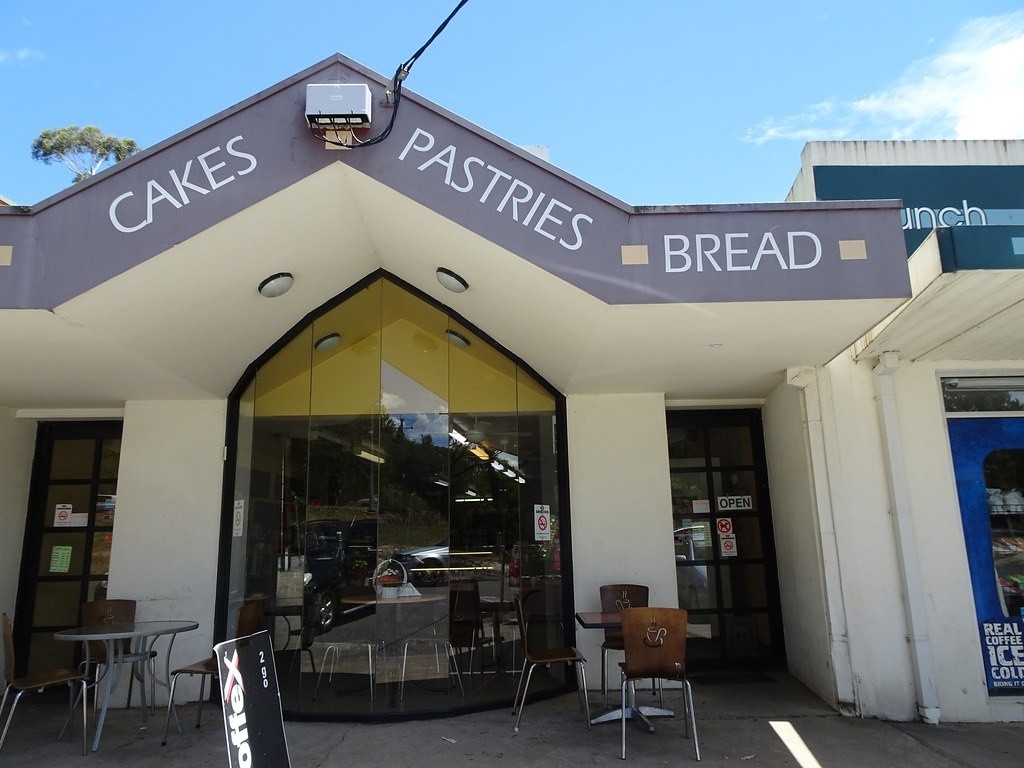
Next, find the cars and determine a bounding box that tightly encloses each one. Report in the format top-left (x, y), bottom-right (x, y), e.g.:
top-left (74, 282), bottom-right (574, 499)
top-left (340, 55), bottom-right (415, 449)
top-left (272, 509), bottom-right (559, 636)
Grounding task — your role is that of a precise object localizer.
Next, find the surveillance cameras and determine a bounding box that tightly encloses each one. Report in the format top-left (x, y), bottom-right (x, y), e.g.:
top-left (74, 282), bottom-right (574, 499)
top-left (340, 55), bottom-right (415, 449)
top-left (946, 378), bottom-right (959, 388)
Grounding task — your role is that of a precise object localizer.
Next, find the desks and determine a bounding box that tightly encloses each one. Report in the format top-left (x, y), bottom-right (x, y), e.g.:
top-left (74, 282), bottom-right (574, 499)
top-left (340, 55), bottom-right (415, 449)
top-left (54, 620), bottom-right (200, 751)
top-left (576, 611), bottom-right (675, 734)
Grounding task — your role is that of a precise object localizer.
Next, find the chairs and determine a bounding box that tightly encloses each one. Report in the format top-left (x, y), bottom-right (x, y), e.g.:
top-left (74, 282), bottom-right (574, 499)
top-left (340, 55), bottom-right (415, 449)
top-left (599, 583), bottom-right (664, 712)
top-left (77, 599), bottom-right (158, 717)
top-left (279, 573), bottom-right (520, 706)
top-left (0, 611), bottom-right (91, 757)
top-left (510, 598), bottom-right (593, 732)
top-left (160, 599), bottom-right (264, 747)
top-left (617, 607), bottom-right (702, 762)
